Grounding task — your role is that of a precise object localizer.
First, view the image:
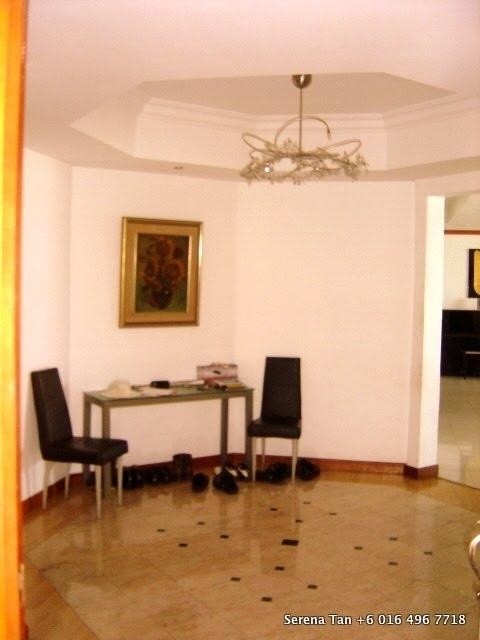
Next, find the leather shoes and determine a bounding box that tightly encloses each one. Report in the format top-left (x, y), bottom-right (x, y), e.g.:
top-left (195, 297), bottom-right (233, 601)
top-left (122, 468), bottom-right (170, 487)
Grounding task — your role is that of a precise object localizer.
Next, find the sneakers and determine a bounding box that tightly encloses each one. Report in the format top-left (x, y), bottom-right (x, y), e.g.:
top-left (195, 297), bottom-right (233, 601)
top-left (191, 458), bottom-right (321, 495)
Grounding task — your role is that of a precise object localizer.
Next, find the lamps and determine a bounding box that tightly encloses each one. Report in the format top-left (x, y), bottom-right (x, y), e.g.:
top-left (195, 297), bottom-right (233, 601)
top-left (240, 72), bottom-right (366, 185)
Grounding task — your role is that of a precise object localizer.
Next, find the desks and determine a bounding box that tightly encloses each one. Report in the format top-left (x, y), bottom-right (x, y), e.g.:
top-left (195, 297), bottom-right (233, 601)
top-left (82, 379), bottom-right (258, 507)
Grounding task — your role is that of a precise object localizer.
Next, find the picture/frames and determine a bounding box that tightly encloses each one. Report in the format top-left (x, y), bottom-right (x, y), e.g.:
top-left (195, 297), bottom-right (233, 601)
top-left (118, 217), bottom-right (203, 329)
top-left (468, 248), bottom-right (479, 298)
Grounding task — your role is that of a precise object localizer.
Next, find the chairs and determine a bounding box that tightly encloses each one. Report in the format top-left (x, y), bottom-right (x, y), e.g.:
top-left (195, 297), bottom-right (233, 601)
top-left (248, 358), bottom-right (302, 484)
top-left (26, 367), bottom-right (130, 518)
